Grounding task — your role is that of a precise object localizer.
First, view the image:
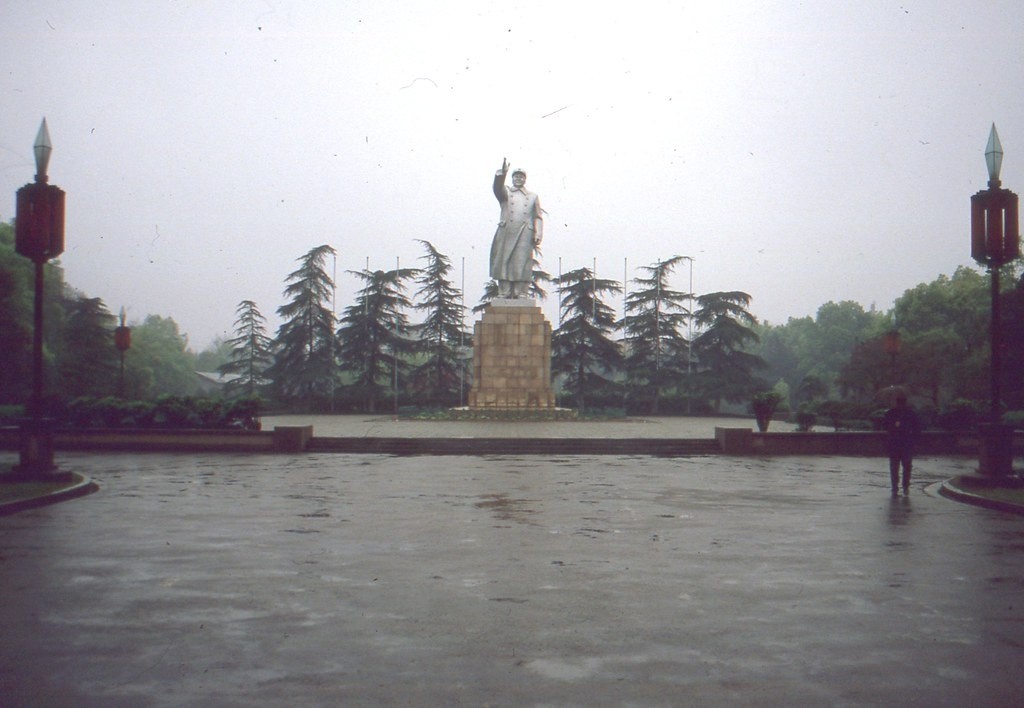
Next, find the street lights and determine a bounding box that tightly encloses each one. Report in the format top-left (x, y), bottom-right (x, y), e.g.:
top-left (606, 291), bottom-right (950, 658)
top-left (114, 305), bottom-right (130, 402)
top-left (960, 122), bottom-right (1024, 489)
top-left (0, 114), bottom-right (70, 482)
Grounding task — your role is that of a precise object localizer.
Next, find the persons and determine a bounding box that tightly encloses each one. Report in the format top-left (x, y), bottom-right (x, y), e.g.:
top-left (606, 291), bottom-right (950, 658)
top-left (491, 156), bottom-right (543, 300)
top-left (882, 396), bottom-right (921, 496)
